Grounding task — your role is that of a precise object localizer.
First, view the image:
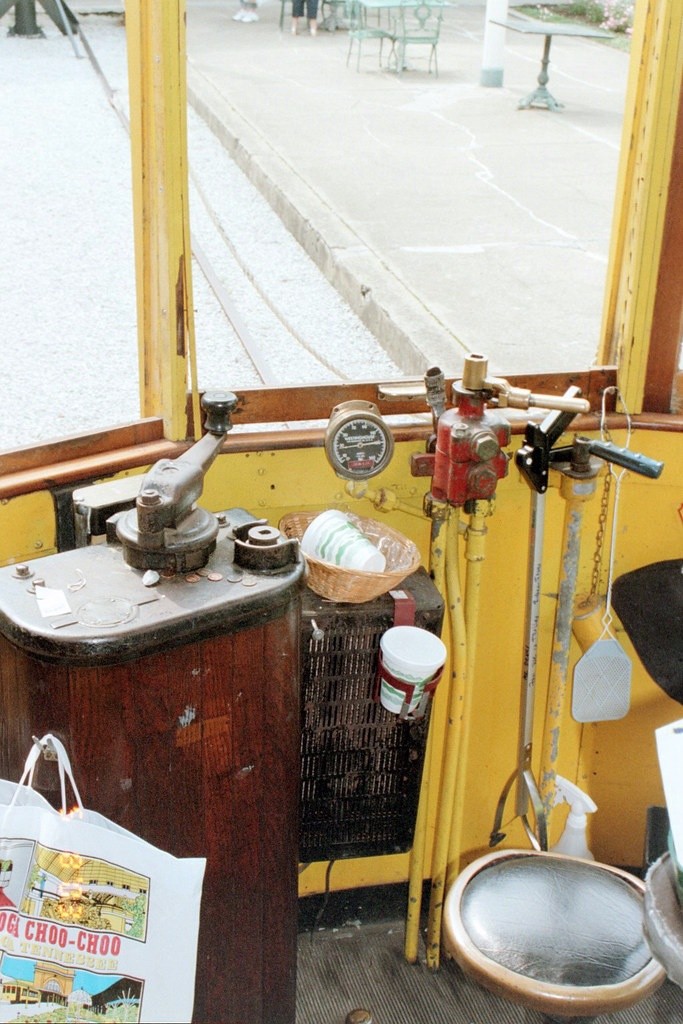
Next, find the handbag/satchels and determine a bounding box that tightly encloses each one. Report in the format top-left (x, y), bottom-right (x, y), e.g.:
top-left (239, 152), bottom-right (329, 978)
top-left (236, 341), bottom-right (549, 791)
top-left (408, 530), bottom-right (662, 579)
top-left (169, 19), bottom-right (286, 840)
top-left (0, 734), bottom-right (207, 1024)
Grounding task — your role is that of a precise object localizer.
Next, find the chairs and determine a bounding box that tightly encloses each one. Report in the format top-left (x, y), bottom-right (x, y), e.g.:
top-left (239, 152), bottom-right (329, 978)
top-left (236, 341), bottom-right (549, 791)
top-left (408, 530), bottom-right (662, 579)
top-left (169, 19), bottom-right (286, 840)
top-left (346, 0), bottom-right (395, 73)
top-left (393, 0), bottom-right (444, 77)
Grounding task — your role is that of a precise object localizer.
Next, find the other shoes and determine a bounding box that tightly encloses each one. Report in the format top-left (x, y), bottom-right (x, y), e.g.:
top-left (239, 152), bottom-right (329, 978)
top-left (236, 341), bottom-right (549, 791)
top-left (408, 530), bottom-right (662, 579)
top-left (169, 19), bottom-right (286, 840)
top-left (232, 9), bottom-right (247, 21)
top-left (292, 19), bottom-right (301, 36)
top-left (309, 19), bottom-right (317, 37)
top-left (240, 11), bottom-right (259, 23)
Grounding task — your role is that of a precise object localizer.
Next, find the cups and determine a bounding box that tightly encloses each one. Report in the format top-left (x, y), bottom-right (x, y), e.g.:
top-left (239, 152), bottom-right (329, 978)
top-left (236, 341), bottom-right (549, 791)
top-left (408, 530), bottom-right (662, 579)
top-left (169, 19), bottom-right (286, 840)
top-left (300, 509), bottom-right (387, 573)
top-left (378, 624), bottom-right (445, 714)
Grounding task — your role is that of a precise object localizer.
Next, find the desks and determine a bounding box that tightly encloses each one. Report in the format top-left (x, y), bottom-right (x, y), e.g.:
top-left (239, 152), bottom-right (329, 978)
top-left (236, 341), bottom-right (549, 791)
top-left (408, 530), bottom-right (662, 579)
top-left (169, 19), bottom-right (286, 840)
top-left (356, 0), bottom-right (449, 38)
top-left (0, 509), bottom-right (303, 1024)
top-left (489, 19), bottom-right (616, 112)
top-left (642, 851), bottom-right (683, 992)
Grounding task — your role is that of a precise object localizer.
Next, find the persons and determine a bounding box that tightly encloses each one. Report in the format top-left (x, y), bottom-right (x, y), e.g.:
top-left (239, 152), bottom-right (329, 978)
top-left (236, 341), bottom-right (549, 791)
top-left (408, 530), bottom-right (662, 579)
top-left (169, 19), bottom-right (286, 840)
top-left (291, 0), bottom-right (317, 34)
top-left (233, 0), bottom-right (259, 21)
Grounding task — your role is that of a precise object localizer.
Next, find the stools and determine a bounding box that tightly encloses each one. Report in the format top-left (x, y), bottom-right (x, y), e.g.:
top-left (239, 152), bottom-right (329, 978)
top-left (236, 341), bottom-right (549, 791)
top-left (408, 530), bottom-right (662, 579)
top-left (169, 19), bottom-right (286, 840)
top-left (443, 848), bottom-right (666, 1024)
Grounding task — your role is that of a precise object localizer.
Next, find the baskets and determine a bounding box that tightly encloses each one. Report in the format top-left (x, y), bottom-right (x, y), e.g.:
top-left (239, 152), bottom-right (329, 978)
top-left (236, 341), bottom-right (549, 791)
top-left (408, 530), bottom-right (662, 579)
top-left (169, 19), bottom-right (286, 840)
top-left (278, 509), bottom-right (421, 603)
